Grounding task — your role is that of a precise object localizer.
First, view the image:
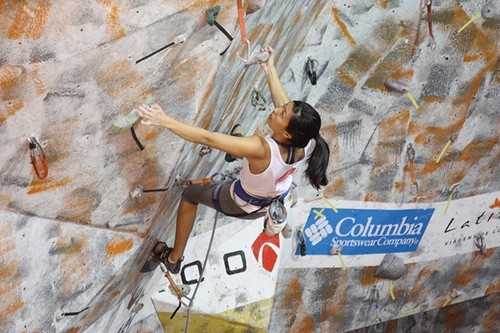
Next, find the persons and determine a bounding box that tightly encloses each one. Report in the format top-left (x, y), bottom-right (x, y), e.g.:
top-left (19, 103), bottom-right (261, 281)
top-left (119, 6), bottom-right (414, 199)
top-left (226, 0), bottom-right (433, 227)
top-left (136, 45), bottom-right (330, 274)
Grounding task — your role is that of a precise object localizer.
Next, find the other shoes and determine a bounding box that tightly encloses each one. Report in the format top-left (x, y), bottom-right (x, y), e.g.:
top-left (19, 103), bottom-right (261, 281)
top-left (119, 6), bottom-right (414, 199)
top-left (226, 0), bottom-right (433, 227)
top-left (153, 240), bottom-right (185, 274)
top-left (225, 123), bottom-right (245, 162)
